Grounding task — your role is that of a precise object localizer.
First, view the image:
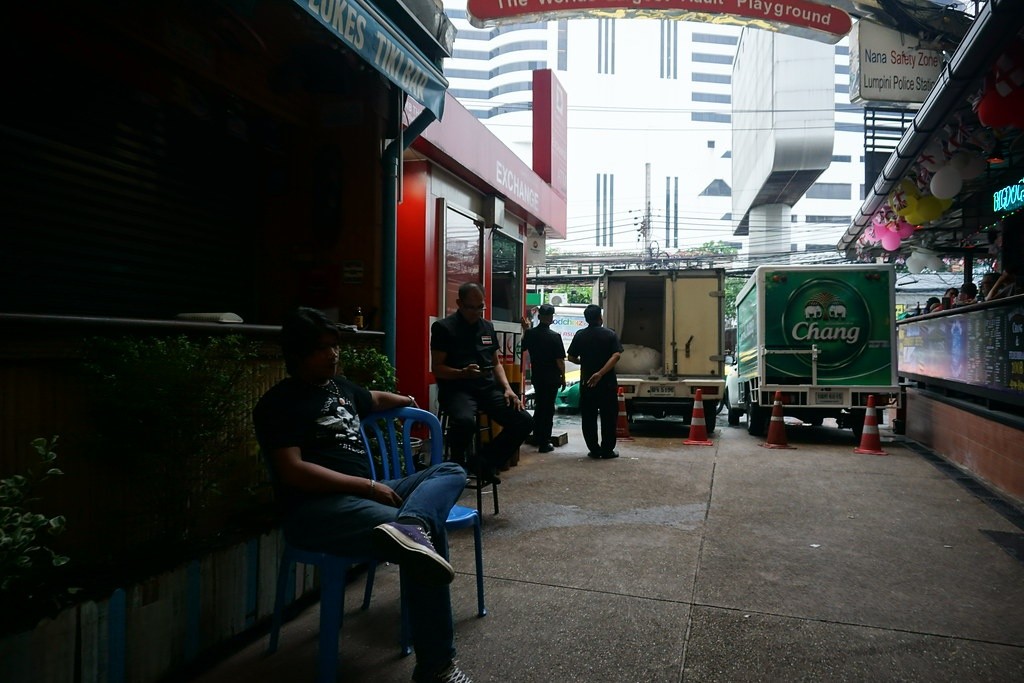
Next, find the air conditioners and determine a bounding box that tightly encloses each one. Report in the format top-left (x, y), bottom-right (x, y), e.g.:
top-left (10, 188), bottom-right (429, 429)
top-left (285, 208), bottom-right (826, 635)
top-left (549, 293), bottom-right (568, 306)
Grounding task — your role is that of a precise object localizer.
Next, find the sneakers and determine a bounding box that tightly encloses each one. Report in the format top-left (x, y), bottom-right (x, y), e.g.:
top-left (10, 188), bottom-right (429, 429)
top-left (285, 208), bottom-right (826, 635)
top-left (411, 658), bottom-right (473, 683)
top-left (373, 521), bottom-right (455, 583)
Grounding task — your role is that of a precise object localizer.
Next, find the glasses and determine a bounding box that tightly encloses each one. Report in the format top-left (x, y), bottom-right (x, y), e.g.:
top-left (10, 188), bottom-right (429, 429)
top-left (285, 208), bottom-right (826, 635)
top-left (461, 303), bottom-right (484, 309)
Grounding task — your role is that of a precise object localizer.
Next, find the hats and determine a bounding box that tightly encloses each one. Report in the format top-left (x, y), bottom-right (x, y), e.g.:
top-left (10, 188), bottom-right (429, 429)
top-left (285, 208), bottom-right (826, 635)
top-left (539, 304), bottom-right (554, 314)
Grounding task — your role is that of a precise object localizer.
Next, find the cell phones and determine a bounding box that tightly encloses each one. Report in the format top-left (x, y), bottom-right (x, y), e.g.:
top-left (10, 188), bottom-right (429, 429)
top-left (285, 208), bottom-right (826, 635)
top-left (482, 366), bottom-right (495, 371)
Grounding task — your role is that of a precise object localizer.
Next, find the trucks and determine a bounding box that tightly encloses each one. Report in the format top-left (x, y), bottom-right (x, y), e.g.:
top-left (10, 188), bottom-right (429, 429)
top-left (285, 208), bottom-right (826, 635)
top-left (592, 260), bottom-right (737, 436)
top-left (724, 263), bottom-right (902, 437)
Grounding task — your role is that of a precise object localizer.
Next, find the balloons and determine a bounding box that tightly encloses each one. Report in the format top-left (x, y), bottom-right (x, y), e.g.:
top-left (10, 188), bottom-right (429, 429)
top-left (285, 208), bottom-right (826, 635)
top-left (865, 90), bottom-right (1024, 273)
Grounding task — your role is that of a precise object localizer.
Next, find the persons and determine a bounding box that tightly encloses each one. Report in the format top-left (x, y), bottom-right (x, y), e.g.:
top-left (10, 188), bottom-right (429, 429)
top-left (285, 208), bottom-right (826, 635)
top-left (567, 304), bottom-right (625, 459)
top-left (251, 304), bottom-right (468, 683)
top-left (923, 268), bottom-right (1007, 315)
top-left (521, 304), bottom-right (566, 453)
top-left (432, 282), bottom-right (535, 485)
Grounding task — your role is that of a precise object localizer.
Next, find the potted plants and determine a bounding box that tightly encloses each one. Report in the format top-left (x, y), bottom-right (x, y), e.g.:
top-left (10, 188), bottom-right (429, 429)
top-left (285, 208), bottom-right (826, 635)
top-left (0, 332), bottom-right (403, 683)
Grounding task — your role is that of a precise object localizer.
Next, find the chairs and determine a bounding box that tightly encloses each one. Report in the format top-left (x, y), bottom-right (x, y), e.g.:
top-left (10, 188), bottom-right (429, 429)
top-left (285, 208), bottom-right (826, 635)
top-left (438, 407), bottom-right (499, 526)
top-left (268, 404), bottom-right (486, 683)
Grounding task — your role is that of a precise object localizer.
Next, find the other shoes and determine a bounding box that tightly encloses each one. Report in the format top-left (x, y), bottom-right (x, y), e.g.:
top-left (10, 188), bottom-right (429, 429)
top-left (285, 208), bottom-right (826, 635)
top-left (468, 454), bottom-right (501, 486)
top-left (587, 451), bottom-right (600, 459)
top-left (448, 450), bottom-right (469, 476)
top-left (538, 443), bottom-right (554, 453)
top-left (600, 451), bottom-right (619, 458)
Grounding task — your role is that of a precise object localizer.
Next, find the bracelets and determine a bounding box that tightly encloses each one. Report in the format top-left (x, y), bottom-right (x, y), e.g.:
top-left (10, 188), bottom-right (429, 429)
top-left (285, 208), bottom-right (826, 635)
top-left (599, 368), bottom-right (607, 375)
top-left (408, 395), bottom-right (415, 406)
top-left (370, 480), bottom-right (376, 500)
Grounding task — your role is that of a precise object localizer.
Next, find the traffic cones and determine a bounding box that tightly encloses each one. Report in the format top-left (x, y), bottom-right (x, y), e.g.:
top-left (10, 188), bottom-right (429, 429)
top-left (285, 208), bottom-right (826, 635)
top-left (682, 388), bottom-right (714, 446)
top-left (760, 390), bottom-right (799, 450)
top-left (614, 388), bottom-right (635, 442)
top-left (853, 395), bottom-right (890, 456)
